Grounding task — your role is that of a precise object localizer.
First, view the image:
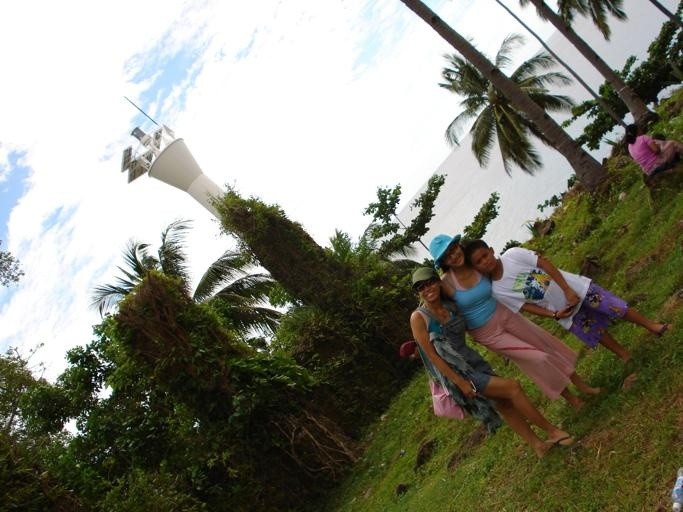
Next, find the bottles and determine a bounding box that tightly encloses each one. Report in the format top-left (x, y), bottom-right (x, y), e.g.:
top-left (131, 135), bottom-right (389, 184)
top-left (670, 465), bottom-right (683, 512)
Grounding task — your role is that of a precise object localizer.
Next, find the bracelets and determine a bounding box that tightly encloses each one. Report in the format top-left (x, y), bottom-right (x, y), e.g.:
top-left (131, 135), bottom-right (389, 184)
top-left (553, 310), bottom-right (560, 322)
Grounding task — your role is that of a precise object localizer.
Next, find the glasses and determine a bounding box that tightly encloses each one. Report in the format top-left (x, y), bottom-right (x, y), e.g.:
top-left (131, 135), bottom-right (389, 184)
top-left (413, 276), bottom-right (439, 292)
top-left (439, 241), bottom-right (459, 263)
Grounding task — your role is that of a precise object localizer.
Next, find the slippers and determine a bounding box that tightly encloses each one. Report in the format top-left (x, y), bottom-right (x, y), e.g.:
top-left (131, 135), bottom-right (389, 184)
top-left (540, 433), bottom-right (575, 459)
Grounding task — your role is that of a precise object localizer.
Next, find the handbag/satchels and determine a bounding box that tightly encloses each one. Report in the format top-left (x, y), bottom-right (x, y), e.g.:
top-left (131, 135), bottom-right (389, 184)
top-left (429, 378), bottom-right (465, 420)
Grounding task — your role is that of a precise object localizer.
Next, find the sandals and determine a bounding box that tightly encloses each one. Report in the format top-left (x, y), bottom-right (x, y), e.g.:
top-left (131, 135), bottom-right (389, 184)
top-left (623, 359), bottom-right (635, 371)
top-left (656, 323), bottom-right (673, 338)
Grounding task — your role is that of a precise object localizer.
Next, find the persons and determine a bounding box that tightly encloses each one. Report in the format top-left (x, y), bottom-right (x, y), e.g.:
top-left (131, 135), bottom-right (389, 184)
top-left (465, 237), bottom-right (675, 369)
top-left (425, 232), bottom-right (606, 415)
top-left (408, 266), bottom-right (575, 460)
top-left (624, 124), bottom-right (683, 176)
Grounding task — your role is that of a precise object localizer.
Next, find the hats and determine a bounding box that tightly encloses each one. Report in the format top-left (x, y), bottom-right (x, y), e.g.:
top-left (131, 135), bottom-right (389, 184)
top-left (428, 234), bottom-right (461, 270)
top-left (412, 267), bottom-right (438, 286)
